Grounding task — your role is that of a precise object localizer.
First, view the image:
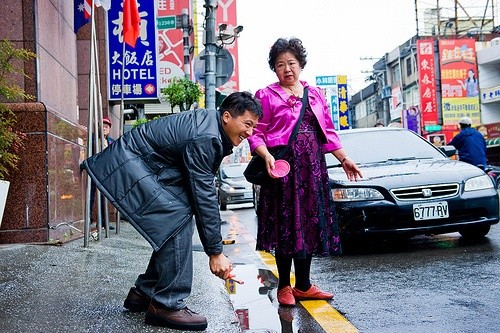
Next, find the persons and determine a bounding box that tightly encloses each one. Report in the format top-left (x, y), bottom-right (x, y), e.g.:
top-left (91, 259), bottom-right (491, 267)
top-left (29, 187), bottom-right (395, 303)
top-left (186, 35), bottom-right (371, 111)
top-left (457, 70), bottom-right (478, 97)
top-left (84, 92), bottom-right (263, 330)
top-left (90, 117), bottom-right (117, 230)
top-left (246, 37), bottom-right (363, 307)
top-left (446, 119), bottom-right (489, 173)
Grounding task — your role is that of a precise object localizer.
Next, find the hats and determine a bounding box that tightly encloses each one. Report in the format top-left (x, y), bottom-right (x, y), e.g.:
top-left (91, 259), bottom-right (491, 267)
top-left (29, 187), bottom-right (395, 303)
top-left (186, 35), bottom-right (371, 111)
top-left (103, 119), bottom-right (111, 128)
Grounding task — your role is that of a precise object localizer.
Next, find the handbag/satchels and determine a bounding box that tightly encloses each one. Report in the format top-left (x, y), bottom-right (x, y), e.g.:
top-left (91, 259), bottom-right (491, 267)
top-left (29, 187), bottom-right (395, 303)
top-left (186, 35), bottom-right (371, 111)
top-left (243, 144), bottom-right (292, 186)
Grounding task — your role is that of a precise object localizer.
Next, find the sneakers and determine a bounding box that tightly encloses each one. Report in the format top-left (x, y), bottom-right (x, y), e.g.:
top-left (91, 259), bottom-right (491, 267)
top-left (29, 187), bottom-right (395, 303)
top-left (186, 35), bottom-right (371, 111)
top-left (145, 300), bottom-right (207, 331)
top-left (123, 287), bottom-right (151, 312)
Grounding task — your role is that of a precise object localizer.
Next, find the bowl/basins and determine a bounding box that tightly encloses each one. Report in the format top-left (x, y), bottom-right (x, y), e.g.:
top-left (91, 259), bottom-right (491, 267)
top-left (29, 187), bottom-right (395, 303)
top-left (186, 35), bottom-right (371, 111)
top-left (270, 159), bottom-right (291, 177)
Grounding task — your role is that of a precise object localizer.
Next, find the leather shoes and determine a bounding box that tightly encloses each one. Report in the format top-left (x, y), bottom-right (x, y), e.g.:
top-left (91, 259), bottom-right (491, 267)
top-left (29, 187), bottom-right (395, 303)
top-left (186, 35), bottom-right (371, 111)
top-left (292, 284), bottom-right (334, 299)
top-left (277, 286), bottom-right (296, 307)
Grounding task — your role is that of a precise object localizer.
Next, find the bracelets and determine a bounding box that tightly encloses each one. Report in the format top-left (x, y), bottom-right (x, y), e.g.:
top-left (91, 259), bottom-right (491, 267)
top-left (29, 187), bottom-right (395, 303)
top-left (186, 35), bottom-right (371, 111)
top-left (340, 155), bottom-right (348, 163)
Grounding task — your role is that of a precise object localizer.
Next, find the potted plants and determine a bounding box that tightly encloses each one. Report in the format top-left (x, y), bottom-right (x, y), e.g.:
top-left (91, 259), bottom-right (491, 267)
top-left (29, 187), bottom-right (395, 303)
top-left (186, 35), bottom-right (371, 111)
top-left (0, 38), bottom-right (38, 228)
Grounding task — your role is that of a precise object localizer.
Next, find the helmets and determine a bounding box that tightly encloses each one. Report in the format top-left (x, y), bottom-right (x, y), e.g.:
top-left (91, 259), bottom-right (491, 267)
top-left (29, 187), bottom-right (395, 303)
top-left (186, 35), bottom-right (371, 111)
top-left (459, 117), bottom-right (472, 125)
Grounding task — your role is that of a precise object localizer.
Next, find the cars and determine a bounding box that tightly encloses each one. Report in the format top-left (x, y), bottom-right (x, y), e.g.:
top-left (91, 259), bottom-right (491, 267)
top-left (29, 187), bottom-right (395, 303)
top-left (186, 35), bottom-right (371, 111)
top-left (252, 126), bottom-right (500, 257)
top-left (214, 162), bottom-right (254, 211)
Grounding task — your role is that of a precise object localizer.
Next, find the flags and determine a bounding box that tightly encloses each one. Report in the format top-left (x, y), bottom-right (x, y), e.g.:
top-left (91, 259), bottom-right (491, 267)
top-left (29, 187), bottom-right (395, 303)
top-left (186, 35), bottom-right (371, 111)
top-left (123, 0), bottom-right (140, 47)
top-left (73, 0), bottom-right (94, 34)
